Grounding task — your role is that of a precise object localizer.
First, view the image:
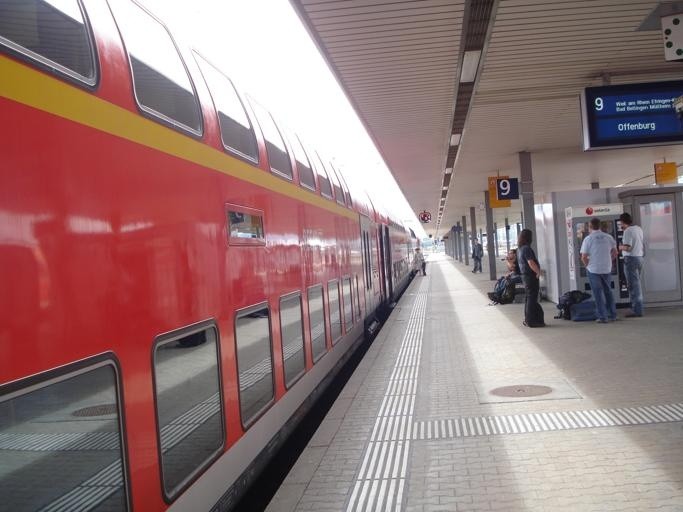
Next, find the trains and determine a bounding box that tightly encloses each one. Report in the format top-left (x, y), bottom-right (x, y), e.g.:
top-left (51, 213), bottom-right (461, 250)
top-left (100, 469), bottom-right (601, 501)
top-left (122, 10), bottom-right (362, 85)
top-left (0, 0), bottom-right (422, 511)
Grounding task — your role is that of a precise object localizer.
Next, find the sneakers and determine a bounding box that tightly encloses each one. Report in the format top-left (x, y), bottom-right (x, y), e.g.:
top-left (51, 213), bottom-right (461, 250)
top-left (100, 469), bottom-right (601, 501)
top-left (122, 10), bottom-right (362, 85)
top-left (625, 311), bottom-right (643, 318)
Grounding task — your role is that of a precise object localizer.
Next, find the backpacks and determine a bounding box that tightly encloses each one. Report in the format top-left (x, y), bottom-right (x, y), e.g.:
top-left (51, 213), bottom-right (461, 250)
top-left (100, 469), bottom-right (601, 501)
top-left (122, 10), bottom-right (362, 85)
top-left (487, 274), bottom-right (516, 307)
top-left (557, 288), bottom-right (591, 308)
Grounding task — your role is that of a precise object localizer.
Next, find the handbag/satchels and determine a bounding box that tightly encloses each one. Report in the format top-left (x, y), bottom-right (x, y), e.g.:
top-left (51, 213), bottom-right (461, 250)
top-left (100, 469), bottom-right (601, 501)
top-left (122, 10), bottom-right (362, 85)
top-left (570, 301), bottom-right (597, 321)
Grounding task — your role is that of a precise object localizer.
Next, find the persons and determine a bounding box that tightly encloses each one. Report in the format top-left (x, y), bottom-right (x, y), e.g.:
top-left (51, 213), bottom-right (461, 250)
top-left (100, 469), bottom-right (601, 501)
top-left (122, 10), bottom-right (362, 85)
top-left (470, 238), bottom-right (483, 273)
top-left (615, 211), bottom-right (646, 318)
top-left (413, 247), bottom-right (426, 276)
top-left (578, 217), bottom-right (619, 324)
top-left (515, 228), bottom-right (546, 328)
top-left (485, 249), bottom-right (521, 304)
